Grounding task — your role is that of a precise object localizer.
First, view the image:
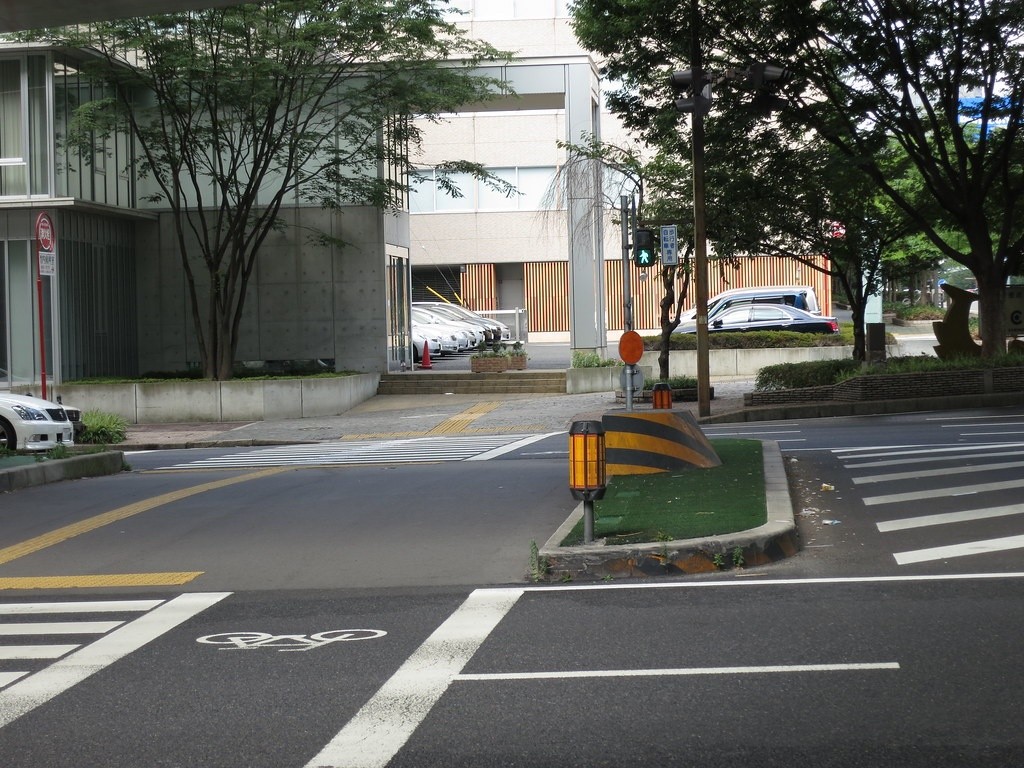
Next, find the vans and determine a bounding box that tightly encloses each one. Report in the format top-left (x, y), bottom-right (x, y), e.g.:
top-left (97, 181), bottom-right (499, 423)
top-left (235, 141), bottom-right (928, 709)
top-left (669, 285), bottom-right (824, 323)
top-left (691, 290), bottom-right (813, 321)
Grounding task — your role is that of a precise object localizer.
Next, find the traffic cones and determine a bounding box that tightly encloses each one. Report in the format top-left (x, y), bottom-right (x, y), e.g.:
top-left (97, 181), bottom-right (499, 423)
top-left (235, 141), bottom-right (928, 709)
top-left (416, 340), bottom-right (433, 369)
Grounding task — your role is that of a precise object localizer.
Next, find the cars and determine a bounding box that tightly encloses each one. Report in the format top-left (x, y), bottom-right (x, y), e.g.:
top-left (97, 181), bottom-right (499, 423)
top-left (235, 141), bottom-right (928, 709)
top-left (670, 303), bottom-right (841, 338)
top-left (412, 301), bottom-right (510, 363)
top-left (59, 403), bottom-right (84, 436)
top-left (0, 390), bottom-right (75, 454)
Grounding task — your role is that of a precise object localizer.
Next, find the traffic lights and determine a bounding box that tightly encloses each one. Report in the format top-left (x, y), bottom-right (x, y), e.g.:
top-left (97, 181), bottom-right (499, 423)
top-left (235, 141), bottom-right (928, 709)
top-left (634, 229), bottom-right (657, 268)
